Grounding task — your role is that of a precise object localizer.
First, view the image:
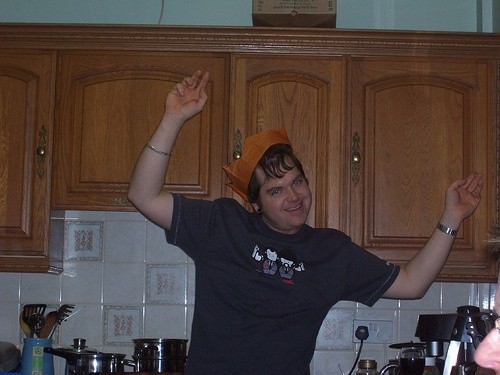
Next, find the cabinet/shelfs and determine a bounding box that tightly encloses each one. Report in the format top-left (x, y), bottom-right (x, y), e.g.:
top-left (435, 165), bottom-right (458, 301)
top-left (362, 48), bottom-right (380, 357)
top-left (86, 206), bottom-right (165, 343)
top-left (50, 50), bottom-right (231, 213)
top-left (1, 48), bottom-right (65, 274)
top-left (347, 55), bottom-right (497, 285)
top-left (230, 55), bottom-right (346, 234)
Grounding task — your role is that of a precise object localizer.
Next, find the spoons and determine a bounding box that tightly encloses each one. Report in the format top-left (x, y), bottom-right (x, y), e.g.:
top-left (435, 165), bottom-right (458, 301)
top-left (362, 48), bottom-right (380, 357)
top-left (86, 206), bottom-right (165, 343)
top-left (27, 313), bottom-right (43, 337)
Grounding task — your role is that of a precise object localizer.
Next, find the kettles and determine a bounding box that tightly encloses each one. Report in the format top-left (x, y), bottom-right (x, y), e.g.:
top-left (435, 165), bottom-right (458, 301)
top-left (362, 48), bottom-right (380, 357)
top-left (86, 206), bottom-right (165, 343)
top-left (442, 306), bottom-right (495, 375)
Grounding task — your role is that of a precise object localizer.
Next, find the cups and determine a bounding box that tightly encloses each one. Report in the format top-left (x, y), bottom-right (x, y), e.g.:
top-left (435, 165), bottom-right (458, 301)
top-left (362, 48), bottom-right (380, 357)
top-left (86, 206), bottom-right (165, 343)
top-left (397, 344), bottom-right (425, 375)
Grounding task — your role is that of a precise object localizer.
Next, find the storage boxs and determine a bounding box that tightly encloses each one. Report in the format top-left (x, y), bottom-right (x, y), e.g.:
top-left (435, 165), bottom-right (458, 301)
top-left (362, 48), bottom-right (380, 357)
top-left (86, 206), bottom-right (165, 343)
top-left (252, 0), bottom-right (336, 29)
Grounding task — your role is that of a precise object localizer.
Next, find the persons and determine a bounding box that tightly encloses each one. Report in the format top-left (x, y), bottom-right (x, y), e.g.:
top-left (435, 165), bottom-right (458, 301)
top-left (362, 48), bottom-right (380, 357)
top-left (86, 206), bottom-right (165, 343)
top-left (473, 220), bottom-right (500, 375)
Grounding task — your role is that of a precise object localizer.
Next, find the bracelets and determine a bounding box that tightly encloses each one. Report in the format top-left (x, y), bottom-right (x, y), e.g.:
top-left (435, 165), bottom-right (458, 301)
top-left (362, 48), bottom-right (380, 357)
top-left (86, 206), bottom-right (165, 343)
top-left (127, 70), bottom-right (483, 375)
top-left (436, 222), bottom-right (458, 236)
top-left (146, 142), bottom-right (171, 157)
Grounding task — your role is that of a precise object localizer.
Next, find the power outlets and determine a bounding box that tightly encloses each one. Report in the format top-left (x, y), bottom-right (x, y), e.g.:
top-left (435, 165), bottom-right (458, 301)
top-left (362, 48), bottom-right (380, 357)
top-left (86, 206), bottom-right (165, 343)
top-left (353, 320), bottom-right (393, 343)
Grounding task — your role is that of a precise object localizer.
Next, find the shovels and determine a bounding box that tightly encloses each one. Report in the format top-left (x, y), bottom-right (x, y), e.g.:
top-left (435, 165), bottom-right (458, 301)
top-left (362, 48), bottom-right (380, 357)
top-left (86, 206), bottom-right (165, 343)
top-left (22, 304), bottom-right (47, 338)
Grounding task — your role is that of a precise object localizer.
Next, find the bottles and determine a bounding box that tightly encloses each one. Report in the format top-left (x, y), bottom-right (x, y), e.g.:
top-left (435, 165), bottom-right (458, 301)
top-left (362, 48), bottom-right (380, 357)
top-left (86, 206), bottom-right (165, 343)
top-left (356, 360), bottom-right (378, 375)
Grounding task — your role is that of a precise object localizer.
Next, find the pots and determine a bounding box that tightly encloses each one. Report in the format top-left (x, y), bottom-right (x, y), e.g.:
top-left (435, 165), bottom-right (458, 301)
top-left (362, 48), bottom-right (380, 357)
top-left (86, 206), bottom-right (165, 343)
top-left (74, 352), bottom-right (125, 375)
top-left (121, 338), bottom-right (189, 375)
top-left (44, 338), bottom-right (95, 375)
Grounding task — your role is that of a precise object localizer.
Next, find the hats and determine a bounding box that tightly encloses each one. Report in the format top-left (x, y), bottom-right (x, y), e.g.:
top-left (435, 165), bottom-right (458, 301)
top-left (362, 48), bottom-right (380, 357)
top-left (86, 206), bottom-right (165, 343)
top-left (222, 127), bottom-right (291, 202)
